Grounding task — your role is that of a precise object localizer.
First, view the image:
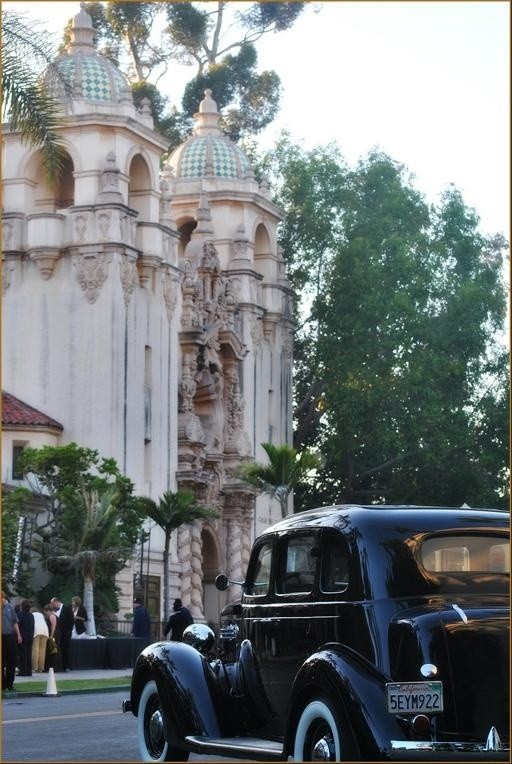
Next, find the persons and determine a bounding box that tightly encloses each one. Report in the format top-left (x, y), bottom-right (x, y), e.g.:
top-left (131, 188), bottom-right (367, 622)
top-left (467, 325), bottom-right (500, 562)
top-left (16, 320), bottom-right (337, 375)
top-left (70, 596), bottom-right (88, 636)
top-left (1, 591), bottom-right (24, 692)
top-left (175, 599), bottom-right (193, 625)
top-left (14, 602), bottom-right (65, 676)
top-left (50, 596), bottom-right (76, 673)
top-left (128, 596), bottom-right (151, 637)
top-left (162, 598), bottom-right (194, 642)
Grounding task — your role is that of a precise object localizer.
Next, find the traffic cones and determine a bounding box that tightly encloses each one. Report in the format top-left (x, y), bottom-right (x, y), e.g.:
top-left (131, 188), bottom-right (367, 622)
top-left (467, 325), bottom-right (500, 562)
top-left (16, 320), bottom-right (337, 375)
top-left (41, 667), bottom-right (61, 697)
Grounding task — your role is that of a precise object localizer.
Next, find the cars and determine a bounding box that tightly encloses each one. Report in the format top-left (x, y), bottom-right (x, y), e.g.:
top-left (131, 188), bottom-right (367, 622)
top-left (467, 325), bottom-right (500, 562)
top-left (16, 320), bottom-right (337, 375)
top-left (123, 503), bottom-right (511, 763)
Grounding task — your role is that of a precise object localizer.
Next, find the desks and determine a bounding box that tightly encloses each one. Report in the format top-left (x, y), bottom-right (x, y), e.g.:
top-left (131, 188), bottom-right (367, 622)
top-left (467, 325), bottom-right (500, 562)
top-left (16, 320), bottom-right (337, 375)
top-left (68, 637), bottom-right (153, 670)
